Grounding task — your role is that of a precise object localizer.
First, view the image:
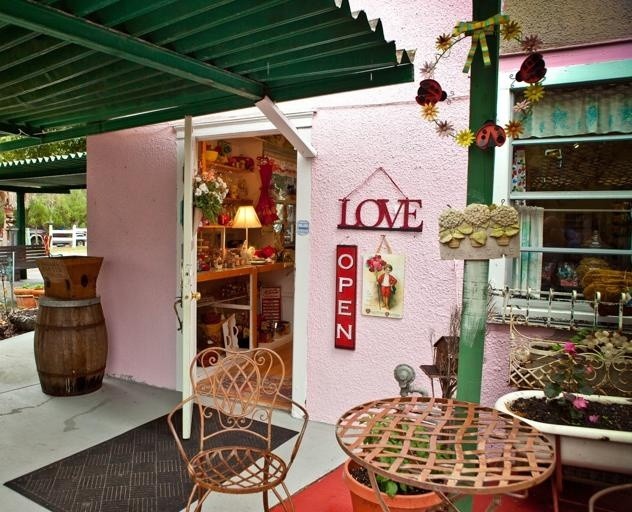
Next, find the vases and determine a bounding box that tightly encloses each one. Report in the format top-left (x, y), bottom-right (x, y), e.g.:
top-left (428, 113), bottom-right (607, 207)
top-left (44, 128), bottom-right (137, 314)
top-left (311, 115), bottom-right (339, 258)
top-left (494, 390), bottom-right (632, 474)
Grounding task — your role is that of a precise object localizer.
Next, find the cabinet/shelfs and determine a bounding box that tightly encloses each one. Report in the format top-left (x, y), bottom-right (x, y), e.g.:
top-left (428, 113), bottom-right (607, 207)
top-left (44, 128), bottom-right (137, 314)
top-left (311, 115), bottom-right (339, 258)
top-left (198, 136), bottom-right (294, 383)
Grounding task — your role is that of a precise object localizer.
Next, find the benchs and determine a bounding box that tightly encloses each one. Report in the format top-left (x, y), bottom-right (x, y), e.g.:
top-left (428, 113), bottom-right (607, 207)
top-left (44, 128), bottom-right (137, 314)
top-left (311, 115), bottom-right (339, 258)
top-left (0, 244), bottom-right (63, 281)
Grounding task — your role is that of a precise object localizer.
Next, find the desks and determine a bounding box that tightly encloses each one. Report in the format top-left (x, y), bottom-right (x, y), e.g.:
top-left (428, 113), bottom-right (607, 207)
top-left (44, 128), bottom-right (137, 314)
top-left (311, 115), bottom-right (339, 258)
top-left (335, 396), bottom-right (557, 511)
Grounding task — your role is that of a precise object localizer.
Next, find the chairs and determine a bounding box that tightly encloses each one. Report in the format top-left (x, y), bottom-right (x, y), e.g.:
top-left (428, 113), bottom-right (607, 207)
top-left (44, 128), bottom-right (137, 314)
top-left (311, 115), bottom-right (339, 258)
top-left (589, 484), bottom-right (632, 512)
top-left (167, 347), bottom-right (309, 512)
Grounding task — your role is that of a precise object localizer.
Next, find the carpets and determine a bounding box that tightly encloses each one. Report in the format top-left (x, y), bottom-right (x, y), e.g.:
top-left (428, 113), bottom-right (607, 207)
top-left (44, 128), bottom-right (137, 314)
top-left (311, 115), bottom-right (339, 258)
top-left (3, 402), bottom-right (299, 512)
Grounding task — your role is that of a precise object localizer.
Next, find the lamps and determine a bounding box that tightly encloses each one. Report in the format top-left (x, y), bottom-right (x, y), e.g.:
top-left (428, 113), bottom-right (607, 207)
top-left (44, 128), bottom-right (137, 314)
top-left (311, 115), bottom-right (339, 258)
top-left (232, 206), bottom-right (262, 257)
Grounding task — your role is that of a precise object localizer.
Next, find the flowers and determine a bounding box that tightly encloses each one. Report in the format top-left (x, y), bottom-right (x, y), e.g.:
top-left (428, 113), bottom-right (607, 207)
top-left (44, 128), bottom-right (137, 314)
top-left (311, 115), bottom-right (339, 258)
top-left (415, 14), bottom-right (547, 152)
top-left (367, 256), bottom-right (387, 310)
top-left (192, 172), bottom-right (229, 225)
top-left (543, 342), bottom-right (600, 427)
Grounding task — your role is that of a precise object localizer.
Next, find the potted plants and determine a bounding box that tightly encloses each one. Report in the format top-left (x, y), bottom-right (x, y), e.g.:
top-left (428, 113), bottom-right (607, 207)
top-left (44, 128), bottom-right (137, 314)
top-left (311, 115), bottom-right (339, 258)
top-left (31, 284), bottom-right (45, 308)
top-left (13, 284), bottom-right (36, 308)
top-left (342, 415), bottom-right (448, 512)
